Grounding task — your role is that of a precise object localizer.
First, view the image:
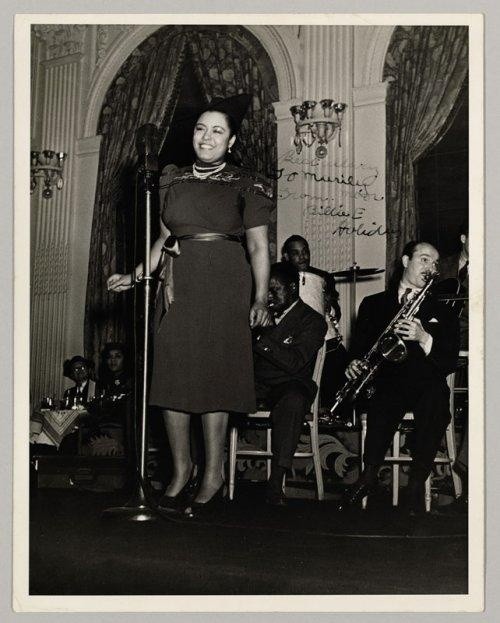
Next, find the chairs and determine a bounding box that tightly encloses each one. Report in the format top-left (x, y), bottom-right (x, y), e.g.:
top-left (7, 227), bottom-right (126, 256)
top-left (230, 340), bottom-right (327, 502)
top-left (360, 350), bottom-right (464, 512)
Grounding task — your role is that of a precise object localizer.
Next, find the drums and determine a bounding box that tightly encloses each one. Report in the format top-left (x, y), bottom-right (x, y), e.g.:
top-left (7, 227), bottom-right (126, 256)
top-left (321, 312), bottom-right (344, 354)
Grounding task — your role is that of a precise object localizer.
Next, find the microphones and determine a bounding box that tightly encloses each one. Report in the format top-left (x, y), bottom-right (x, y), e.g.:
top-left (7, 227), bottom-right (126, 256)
top-left (135, 121), bottom-right (160, 174)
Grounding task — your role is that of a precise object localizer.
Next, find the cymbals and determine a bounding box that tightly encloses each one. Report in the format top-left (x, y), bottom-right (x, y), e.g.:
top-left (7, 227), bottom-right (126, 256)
top-left (324, 267), bottom-right (384, 276)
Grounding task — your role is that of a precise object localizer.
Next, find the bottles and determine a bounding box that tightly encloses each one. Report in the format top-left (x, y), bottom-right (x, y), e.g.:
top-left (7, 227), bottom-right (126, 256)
top-left (65, 387), bottom-right (83, 410)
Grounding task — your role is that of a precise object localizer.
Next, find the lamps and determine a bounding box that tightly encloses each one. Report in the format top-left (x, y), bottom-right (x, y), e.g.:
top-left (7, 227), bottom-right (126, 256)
top-left (290, 99), bottom-right (347, 157)
top-left (30, 149), bottom-right (67, 199)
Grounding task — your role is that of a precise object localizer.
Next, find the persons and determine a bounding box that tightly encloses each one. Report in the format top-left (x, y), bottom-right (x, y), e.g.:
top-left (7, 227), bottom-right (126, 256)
top-left (48, 355), bottom-right (96, 471)
top-left (100, 341), bottom-right (133, 386)
top-left (280, 234), bottom-right (349, 419)
top-left (336, 238), bottom-right (461, 516)
top-left (434, 426), bottom-right (468, 514)
top-left (104, 91), bottom-right (275, 512)
top-left (185, 261), bottom-right (328, 506)
top-left (439, 224), bottom-right (469, 320)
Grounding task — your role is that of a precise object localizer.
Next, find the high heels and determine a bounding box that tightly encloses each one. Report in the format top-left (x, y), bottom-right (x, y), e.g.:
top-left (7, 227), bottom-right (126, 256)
top-left (158, 461), bottom-right (227, 519)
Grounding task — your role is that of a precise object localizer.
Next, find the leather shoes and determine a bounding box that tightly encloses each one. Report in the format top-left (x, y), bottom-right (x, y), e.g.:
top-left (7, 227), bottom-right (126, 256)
top-left (408, 491), bottom-right (426, 515)
top-left (436, 494), bottom-right (468, 514)
top-left (335, 482), bottom-right (367, 513)
top-left (265, 488), bottom-right (289, 506)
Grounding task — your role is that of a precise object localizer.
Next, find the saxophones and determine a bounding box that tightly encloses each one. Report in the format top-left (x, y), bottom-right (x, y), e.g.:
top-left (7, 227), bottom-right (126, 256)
top-left (336, 270), bottom-right (441, 404)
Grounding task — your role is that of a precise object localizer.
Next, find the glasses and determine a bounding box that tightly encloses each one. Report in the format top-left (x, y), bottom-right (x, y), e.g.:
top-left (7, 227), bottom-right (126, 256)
top-left (71, 366), bottom-right (88, 373)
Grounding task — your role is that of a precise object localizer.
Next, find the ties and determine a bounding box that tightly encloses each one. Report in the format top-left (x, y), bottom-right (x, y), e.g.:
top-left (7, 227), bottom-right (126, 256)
top-left (400, 288), bottom-right (412, 305)
